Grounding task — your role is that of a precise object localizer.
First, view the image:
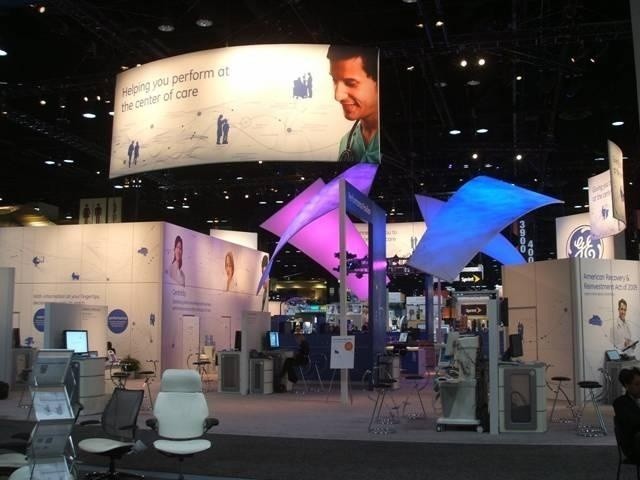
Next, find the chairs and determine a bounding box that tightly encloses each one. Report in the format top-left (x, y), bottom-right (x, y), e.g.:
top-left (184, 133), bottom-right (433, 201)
top-left (145, 367), bottom-right (219, 480)
top-left (75, 386), bottom-right (143, 480)
top-left (610, 416), bottom-right (640, 480)
top-left (292, 350), bottom-right (327, 394)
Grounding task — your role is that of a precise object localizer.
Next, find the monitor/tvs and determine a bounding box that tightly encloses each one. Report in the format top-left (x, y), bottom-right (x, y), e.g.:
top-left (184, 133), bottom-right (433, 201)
top-left (65, 330), bottom-right (88, 354)
top-left (398, 332), bottom-right (408, 342)
top-left (267, 331), bottom-right (280, 349)
top-left (13, 327), bottom-right (20, 347)
top-left (235, 331), bottom-right (240, 347)
top-left (500, 296), bottom-right (508, 326)
top-left (510, 335), bottom-right (524, 357)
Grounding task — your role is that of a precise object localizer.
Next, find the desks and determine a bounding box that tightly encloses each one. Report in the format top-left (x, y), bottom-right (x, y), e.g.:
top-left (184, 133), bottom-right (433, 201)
top-left (216, 351), bottom-right (242, 395)
top-left (251, 358), bottom-right (275, 396)
top-left (604, 360), bottom-right (639, 407)
top-left (70, 357), bottom-right (108, 415)
top-left (499, 363), bottom-right (549, 434)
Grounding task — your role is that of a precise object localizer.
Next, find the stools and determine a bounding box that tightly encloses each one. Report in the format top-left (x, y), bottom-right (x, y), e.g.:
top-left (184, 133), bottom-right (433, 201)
top-left (547, 373), bottom-right (610, 438)
top-left (358, 364), bottom-right (432, 435)
top-left (111, 371), bottom-right (129, 389)
top-left (193, 360), bottom-right (213, 393)
top-left (136, 370), bottom-right (157, 408)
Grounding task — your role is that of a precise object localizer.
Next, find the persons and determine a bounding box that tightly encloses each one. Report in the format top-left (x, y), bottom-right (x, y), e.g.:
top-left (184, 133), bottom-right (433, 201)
top-left (225, 252), bottom-right (238, 291)
top-left (613, 366), bottom-right (640, 466)
top-left (610, 299), bottom-right (635, 359)
top-left (260, 256), bottom-right (268, 311)
top-left (169, 236), bottom-right (185, 286)
top-left (326, 45), bottom-right (378, 162)
